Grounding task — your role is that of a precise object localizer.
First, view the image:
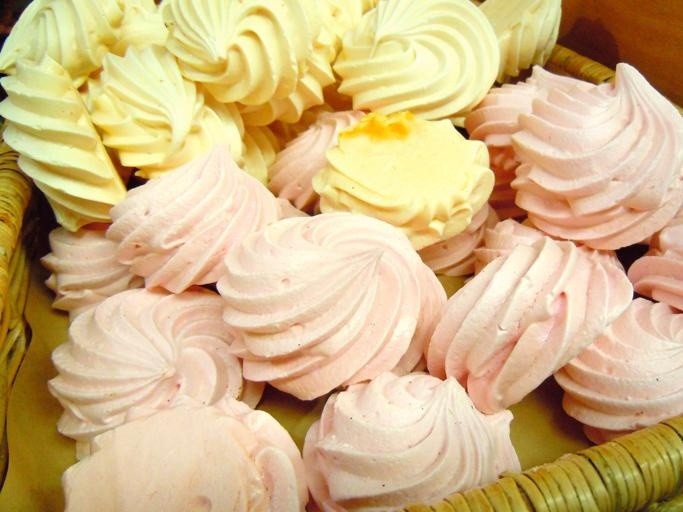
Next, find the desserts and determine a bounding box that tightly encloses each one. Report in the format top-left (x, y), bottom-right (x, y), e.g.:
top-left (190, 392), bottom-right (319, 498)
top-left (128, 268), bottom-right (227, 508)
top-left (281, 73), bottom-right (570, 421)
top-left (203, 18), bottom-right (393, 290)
top-left (0, 0), bottom-right (683, 512)
